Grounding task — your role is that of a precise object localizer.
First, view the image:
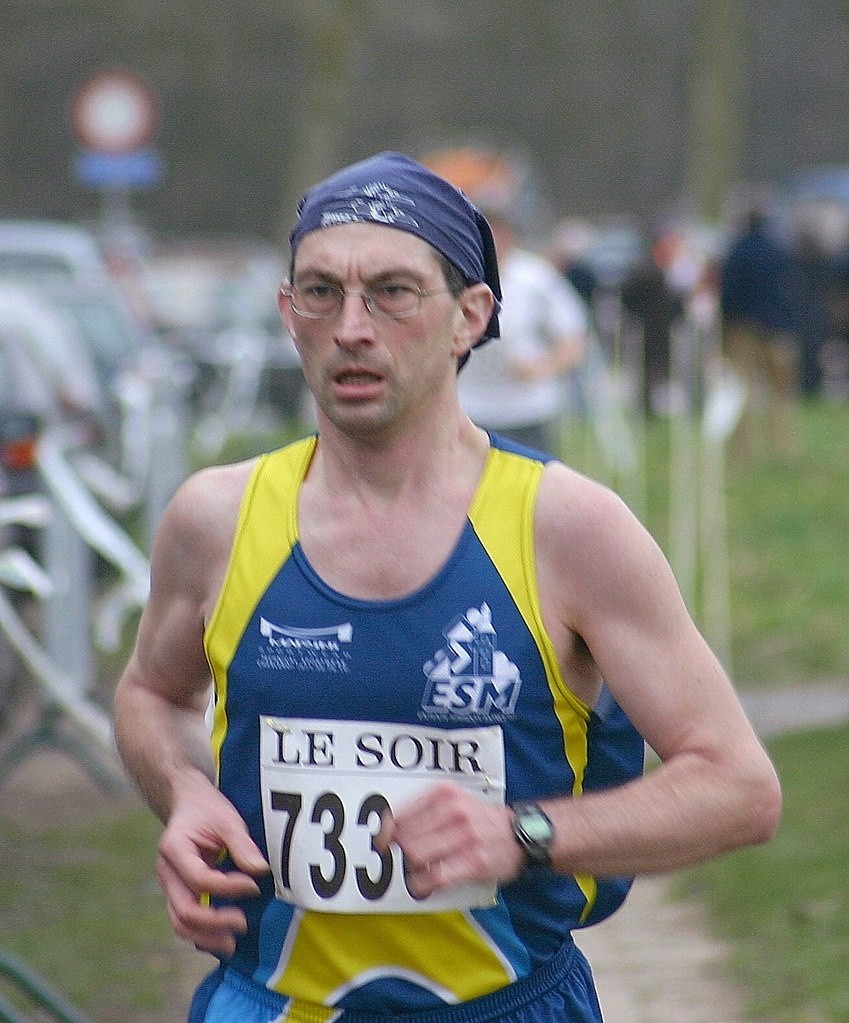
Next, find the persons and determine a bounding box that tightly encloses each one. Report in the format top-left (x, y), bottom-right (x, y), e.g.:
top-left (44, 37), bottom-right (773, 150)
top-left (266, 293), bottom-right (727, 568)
top-left (456, 203), bottom-right (848, 460)
top-left (111, 149), bottom-right (782, 1023)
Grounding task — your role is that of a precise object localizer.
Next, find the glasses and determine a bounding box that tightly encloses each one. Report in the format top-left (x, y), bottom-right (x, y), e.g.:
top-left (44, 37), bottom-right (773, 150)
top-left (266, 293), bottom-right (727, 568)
top-left (283, 281), bottom-right (468, 320)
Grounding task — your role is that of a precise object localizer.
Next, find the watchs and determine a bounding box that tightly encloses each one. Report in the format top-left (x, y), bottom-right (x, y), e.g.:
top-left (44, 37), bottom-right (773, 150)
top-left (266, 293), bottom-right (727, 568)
top-left (507, 799), bottom-right (556, 883)
